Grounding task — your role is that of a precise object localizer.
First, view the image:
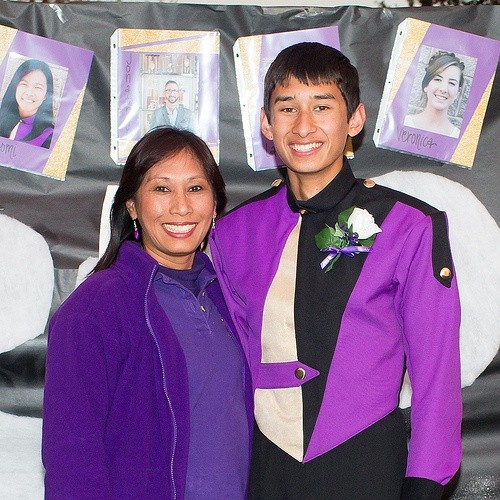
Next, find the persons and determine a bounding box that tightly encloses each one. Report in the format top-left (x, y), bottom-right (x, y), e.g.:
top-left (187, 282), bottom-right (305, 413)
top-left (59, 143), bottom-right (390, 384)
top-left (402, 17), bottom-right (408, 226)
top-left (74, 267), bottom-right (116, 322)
top-left (201, 42), bottom-right (463, 500)
top-left (0, 59), bottom-right (55, 149)
top-left (403, 49), bottom-right (466, 138)
top-left (40, 126), bottom-right (254, 500)
top-left (149, 79), bottom-right (192, 134)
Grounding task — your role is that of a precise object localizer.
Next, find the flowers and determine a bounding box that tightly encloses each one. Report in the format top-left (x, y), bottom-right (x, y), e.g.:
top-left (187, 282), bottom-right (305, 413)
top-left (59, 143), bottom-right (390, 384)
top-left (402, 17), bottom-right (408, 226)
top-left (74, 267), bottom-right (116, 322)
top-left (313, 207), bottom-right (383, 272)
